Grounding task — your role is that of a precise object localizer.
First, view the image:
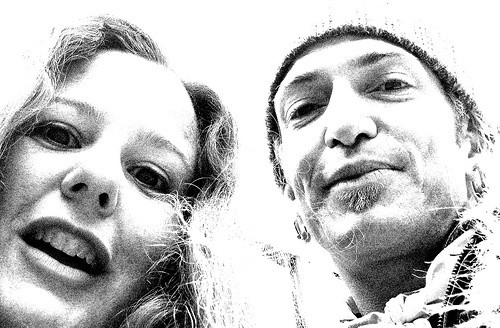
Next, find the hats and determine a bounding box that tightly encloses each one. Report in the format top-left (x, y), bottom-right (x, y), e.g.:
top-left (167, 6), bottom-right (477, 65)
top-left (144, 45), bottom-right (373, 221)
top-left (266, 21), bottom-right (484, 181)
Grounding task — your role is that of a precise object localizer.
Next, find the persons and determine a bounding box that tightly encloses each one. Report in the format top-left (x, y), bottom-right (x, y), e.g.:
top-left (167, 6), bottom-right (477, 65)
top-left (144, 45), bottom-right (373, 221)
top-left (0, 15), bottom-right (242, 328)
top-left (263, 23), bottom-right (500, 328)
